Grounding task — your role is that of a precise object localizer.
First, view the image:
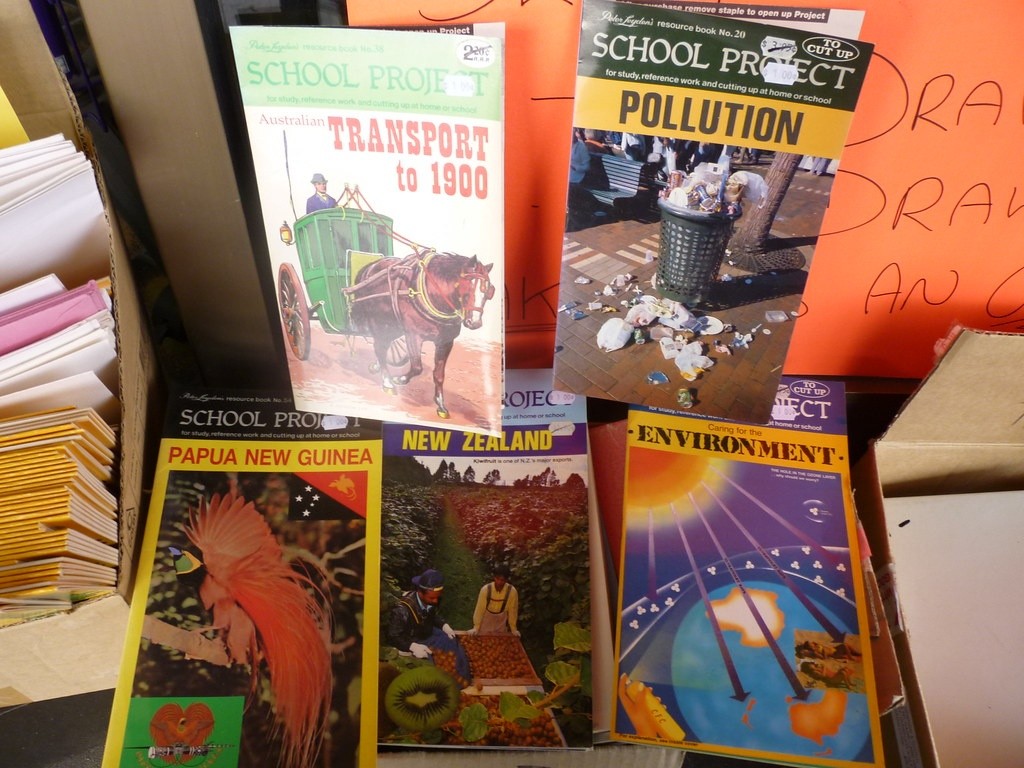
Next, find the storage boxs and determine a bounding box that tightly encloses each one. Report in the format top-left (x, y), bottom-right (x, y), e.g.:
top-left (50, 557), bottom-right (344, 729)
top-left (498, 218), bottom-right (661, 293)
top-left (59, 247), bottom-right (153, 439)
top-left (1, 0), bottom-right (160, 712)
top-left (848, 328), bottom-right (1024, 768)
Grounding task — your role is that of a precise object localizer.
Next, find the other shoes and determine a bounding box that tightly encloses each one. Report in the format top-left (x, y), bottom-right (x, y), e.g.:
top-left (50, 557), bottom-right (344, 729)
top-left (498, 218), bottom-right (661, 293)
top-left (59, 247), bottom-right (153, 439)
top-left (745, 160), bottom-right (758, 165)
top-left (735, 160), bottom-right (744, 164)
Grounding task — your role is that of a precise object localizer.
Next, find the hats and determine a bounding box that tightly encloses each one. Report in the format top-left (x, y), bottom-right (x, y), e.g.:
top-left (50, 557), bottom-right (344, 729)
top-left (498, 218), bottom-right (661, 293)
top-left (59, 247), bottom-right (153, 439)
top-left (412, 569), bottom-right (445, 591)
top-left (310, 173), bottom-right (328, 184)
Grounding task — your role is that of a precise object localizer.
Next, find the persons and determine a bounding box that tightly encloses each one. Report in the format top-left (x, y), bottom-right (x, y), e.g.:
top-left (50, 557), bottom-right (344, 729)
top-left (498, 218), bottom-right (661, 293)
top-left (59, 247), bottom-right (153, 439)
top-left (565, 127), bottom-right (761, 235)
top-left (465, 565), bottom-right (521, 638)
top-left (385, 568), bottom-right (455, 658)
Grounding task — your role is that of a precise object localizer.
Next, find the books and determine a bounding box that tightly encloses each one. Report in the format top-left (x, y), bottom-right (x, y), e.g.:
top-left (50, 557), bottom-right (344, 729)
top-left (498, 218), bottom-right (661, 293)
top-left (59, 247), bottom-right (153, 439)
top-left (100, 364), bottom-right (887, 768)
top-left (0, 132), bottom-right (124, 622)
top-left (229, 24), bottom-right (506, 438)
top-left (554, 1), bottom-right (875, 427)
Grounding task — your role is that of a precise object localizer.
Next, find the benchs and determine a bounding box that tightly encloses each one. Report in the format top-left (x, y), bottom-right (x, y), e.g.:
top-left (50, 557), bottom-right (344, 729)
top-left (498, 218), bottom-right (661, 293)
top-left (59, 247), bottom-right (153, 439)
top-left (581, 151), bottom-right (671, 217)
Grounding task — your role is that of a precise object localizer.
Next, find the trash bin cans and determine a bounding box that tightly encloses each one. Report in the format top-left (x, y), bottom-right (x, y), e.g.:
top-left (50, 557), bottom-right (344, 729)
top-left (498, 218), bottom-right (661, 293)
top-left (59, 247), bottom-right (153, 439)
top-left (656, 195), bottom-right (743, 304)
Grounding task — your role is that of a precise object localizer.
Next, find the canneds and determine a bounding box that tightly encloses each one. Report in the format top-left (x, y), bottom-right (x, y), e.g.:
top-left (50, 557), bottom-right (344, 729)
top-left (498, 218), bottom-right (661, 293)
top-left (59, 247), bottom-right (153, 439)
top-left (678, 388), bottom-right (692, 408)
top-left (633, 328), bottom-right (645, 344)
top-left (670, 171), bottom-right (682, 189)
top-left (694, 185), bottom-right (710, 204)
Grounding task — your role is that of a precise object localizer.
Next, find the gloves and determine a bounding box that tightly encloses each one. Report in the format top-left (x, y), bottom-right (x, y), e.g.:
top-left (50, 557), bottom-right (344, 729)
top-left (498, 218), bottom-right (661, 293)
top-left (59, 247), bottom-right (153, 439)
top-left (514, 631), bottom-right (521, 637)
top-left (443, 623), bottom-right (456, 639)
top-left (467, 629), bottom-right (478, 636)
top-left (410, 642), bottom-right (432, 658)
